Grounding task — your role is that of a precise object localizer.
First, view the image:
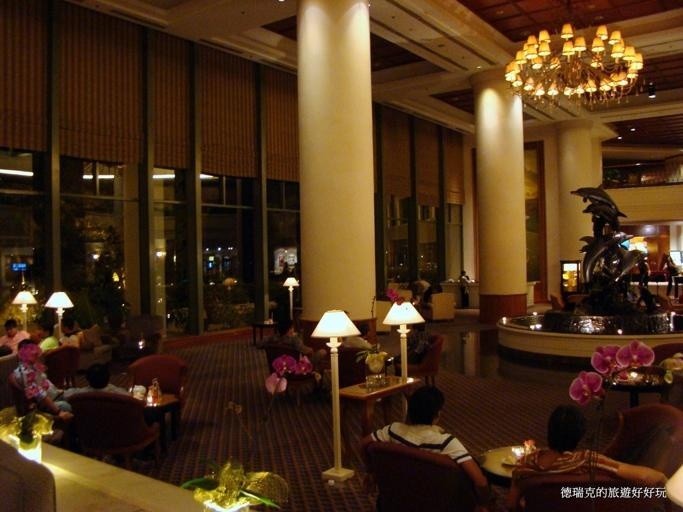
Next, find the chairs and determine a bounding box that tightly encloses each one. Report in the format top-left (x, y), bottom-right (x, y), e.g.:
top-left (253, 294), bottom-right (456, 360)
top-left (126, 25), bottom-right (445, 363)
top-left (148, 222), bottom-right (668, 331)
top-left (515, 472), bottom-right (615, 512)
top-left (368, 441), bottom-right (459, 512)
top-left (396, 289), bottom-right (413, 301)
top-left (602, 402), bottom-right (683, 487)
top-left (0, 353), bottom-right (18, 408)
top-left (257, 341), bottom-right (307, 409)
top-left (42, 345), bottom-right (82, 391)
top-left (385, 334), bottom-right (445, 388)
top-left (113, 314), bottom-right (167, 362)
top-left (67, 391), bottom-right (160, 470)
top-left (422, 292), bottom-right (455, 323)
top-left (127, 354), bottom-right (188, 417)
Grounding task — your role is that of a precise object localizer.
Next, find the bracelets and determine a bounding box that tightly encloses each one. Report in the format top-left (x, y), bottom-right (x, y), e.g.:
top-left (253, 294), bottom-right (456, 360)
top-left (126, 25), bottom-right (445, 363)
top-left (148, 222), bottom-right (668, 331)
top-left (55, 406), bottom-right (63, 417)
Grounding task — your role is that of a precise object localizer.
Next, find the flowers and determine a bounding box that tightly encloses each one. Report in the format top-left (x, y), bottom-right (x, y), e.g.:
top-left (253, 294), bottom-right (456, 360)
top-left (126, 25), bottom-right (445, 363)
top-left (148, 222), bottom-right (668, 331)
top-left (0, 345), bottom-right (60, 446)
top-left (227, 354), bottom-right (311, 473)
top-left (568, 339), bottom-right (655, 483)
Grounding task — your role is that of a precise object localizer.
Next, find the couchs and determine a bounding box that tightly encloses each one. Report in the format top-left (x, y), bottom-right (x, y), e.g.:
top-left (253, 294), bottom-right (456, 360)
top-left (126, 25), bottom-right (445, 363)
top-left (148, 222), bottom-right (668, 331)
top-left (338, 346), bottom-right (376, 389)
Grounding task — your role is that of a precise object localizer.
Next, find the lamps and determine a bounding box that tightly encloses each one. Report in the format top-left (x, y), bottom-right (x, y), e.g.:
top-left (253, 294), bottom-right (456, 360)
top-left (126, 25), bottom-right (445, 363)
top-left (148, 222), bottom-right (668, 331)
top-left (283, 277), bottom-right (299, 321)
top-left (647, 84), bottom-right (657, 99)
top-left (10, 291), bottom-right (37, 333)
top-left (383, 301), bottom-right (425, 423)
top-left (44, 292), bottom-right (74, 339)
top-left (311, 309), bottom-right (361, 484)
top-left (502, 8), bottom-right (644, 115)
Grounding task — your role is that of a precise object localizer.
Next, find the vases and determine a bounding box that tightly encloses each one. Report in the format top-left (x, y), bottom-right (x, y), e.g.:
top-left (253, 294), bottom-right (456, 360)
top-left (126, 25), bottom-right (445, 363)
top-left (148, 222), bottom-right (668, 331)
top-left (15, 436), bottom-right (42, 464)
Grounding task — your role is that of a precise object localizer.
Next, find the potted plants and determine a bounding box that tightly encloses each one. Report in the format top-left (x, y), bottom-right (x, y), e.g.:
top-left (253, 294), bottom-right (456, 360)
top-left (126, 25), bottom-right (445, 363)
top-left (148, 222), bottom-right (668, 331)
top-left (177, 468), bottom-right (290, 512)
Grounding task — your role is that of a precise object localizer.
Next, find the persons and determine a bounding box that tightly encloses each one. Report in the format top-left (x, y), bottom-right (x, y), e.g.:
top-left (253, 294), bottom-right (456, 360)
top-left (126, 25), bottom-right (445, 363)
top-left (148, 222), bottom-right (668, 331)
top-left (342, 320), bottom-right (374, 352)
top-left (269, 274), bottom-right (292, 324)
top-left (459, 269), bottom-right (471, 309)
top-left (31, 316), bottom-right (61, 353)
top-left (406, 279), bottom-right (436, 305)
top-left (14, 338), bottom-right (76, 431)
top-left (0, 345), bottom-right (14, 357)
top-left (358, 384), bottom-right (495, 506)
top-left (0, 318), bottom-right (31, 354)
top-left (57, 315), bottom-right (81, 351)
top-left (63, 363), bottom-right (156, 465)
top-left (260, 318), bottom-right (328, 368)
top-left (382, 322), bottom-right (431, 378)
top-left (72, 319), bottom-right (86, 335)
top-left (503, 403), bottom-right (669, 512)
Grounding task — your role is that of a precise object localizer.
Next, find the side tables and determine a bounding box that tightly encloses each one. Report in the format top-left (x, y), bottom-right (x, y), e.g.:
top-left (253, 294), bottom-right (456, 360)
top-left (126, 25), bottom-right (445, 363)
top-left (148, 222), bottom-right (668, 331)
top-left (250, 318), bottom-right (277, 346)
top-left (330, 375), bottom-right (421, 477)
